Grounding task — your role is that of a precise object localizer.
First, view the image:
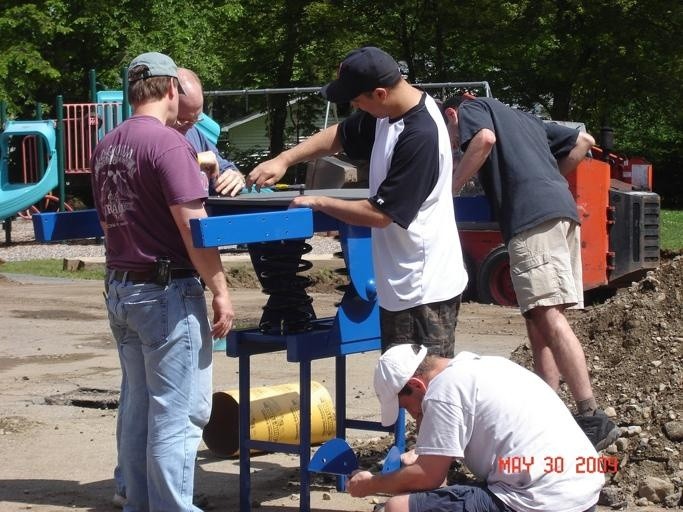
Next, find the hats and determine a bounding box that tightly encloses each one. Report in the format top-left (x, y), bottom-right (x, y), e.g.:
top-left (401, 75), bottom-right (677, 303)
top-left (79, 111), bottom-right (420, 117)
top-left (127, 52), bottom-right (185, 95)
top-left (375, 340), bottom-right (431, 428)
top-left (322, 47), bottom-right (401, 102)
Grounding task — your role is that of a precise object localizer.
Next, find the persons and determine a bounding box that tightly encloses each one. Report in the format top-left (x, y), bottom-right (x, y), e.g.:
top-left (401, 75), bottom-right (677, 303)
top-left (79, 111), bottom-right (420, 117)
top-left (344, 343), bottom-right (605, 512)
top-left (439, 94), bottom-right (621, 451)
top-left (114, 69), bottom-right (245, 507)
top-left (90, 52), bottom-right (234, 512)
top-left (246, 46), bottom-right (469, 441)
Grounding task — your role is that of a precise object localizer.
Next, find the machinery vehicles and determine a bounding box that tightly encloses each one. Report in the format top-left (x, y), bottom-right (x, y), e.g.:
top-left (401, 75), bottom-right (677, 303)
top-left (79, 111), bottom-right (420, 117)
top-left (302, 121), bottom-right (661, 308)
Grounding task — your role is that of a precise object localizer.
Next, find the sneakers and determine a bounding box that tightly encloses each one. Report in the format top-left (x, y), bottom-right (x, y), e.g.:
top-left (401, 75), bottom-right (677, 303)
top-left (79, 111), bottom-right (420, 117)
top-left (575, 408), bottom-right (622, 450)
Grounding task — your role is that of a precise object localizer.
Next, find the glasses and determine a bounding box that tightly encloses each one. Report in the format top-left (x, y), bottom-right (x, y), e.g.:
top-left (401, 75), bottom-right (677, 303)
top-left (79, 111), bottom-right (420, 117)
top-left (177, 112), bottom-right (205, 126)
